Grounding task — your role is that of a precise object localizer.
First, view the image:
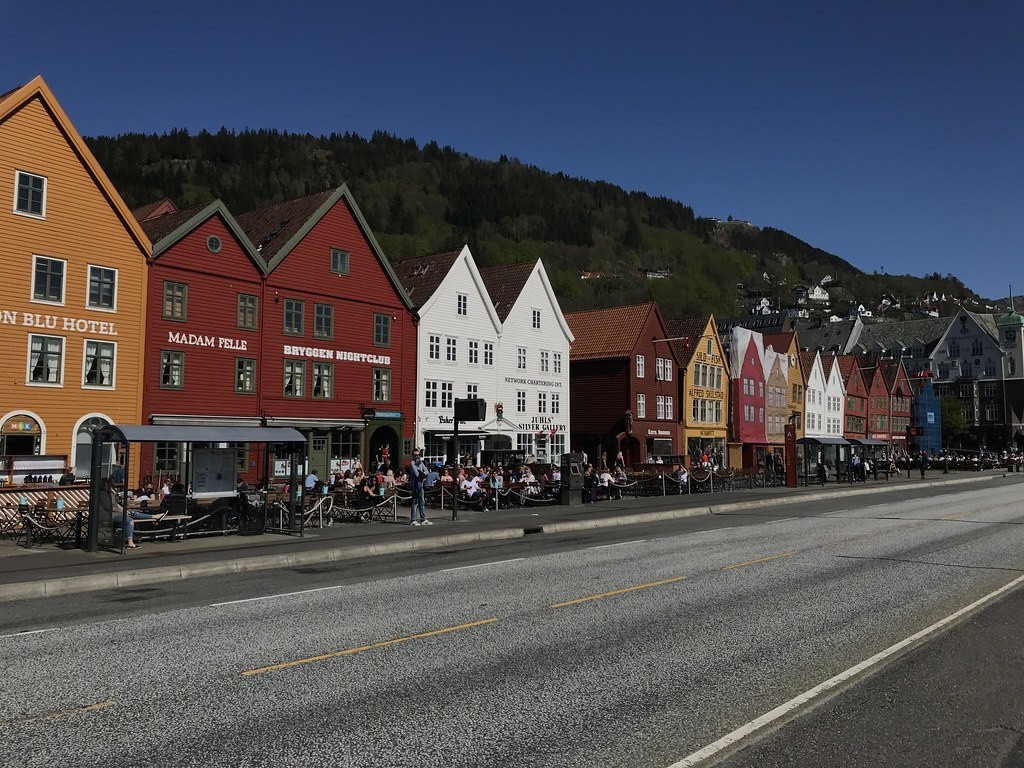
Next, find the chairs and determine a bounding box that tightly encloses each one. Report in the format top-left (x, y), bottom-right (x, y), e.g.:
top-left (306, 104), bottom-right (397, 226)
top-left (0, 476), bottom-right (393, 547)
top-left (586, 463), bottom-right (788, 502)
top-left (931, 454), bottom-right (1024, 470)
top-left (393, 463), bottom-right (562, 509)
top-left (796, 455), bottom-right (931, 485)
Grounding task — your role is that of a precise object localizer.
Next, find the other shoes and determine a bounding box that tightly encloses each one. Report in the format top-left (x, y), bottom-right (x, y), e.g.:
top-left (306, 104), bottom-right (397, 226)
top-left (158, 510), bottom-right (168, 521)
top-left (127, 545), bottom-right (142, 549)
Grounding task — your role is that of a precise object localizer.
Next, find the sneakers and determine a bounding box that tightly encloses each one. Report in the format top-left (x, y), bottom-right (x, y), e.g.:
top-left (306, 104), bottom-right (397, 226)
top-left (421, 520), bottom-right (433, 525)
top-left (411, 520), bottom-right (420, 526)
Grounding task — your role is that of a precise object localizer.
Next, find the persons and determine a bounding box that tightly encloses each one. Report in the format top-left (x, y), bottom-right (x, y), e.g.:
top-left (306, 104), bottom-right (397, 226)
top-left (130, 480), bottom-right (155, 503)
top-left (162, 433), bottom-right (1024, 518)
top-left (159, 477), bottom-right (171, 501)
top-left (101, 477), bottom-right (169, 551)
top-left (409, 448), bottom-right (434, 527)
